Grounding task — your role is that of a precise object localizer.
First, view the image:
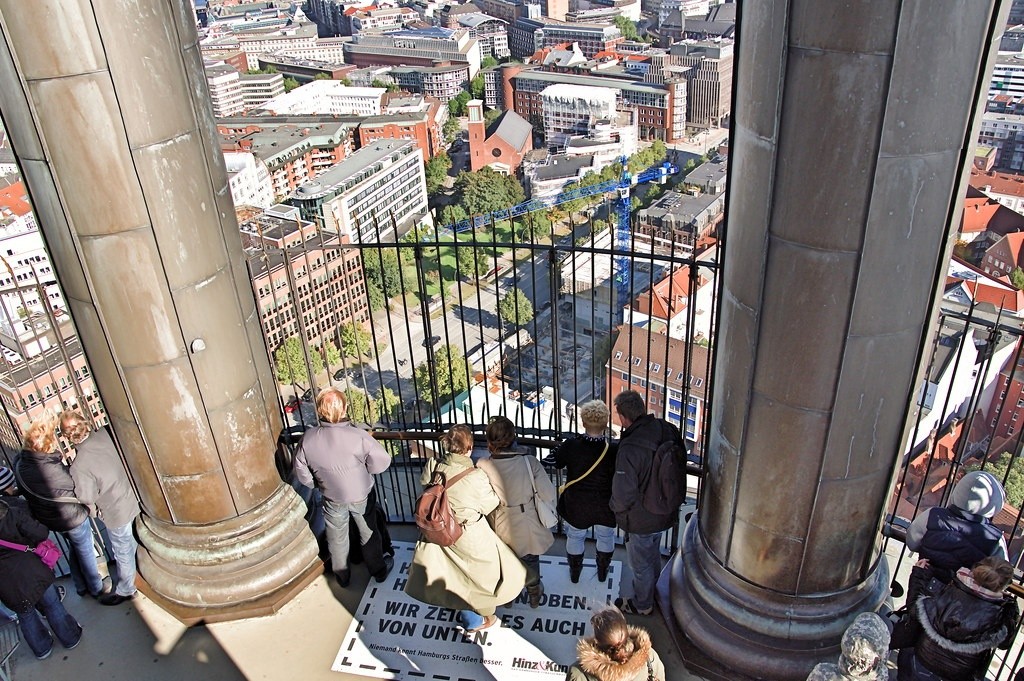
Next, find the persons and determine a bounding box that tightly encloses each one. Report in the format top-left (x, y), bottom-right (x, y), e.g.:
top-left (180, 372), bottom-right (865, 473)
top-left (58, 411), bottom-right (142, 606)
top-left (541, 399), bottom-right (620, 582)
top-left (294, 387), bottom-right (396, 588)
top-left (609, 388), bottom-right (688, 616)
top-left (807, 612), bottom-right (891, 681)
top-left (906, 470), bottom-right (1010, 611)
top-left (0, 466), bottom-right (84, 660)
top-left (477, 415), bottom-right (558, 609)
top-left (565, 610), bottom-right (666, 680)
top-left (403, 423), bottom-right (537, 634)
top-left (14, 421), bottom-right (113, 599)
top-left (896, 555), bottom-right (1024, 681)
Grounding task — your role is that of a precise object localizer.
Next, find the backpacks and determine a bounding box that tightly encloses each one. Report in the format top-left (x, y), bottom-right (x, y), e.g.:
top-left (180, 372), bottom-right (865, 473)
top-left (619, 418), bottom-right (687, 515)
top-left (414, 458), bottom-right (476, 547)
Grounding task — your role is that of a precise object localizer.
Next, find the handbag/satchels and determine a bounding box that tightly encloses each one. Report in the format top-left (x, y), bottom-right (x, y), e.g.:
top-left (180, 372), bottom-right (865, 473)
top-left (33, 537), bottom-right (61, 569)
top-left (524, 454), bottom-right (557, 529)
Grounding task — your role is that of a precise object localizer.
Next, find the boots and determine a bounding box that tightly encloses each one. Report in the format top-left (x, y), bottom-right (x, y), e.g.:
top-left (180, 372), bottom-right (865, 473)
top-left (565, 547), bottom-right (585, 583)
top-left (527, 579), bottom-right (544, 608)
top-left (595, 548), bottom-right (616, 582)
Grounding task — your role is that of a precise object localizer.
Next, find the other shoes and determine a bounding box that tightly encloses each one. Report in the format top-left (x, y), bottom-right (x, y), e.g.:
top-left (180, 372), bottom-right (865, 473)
top-left (376, 555), bottom-right (394, 583)
top-left (78, 588), bottom-right (88, 596)
top-left (65, 621), bottom-right (82, 649)
top-left (100, 594), bottom-right (131, 606)
top-left (37, 629), bottom-right (52, 660)
top-left (338, 581), bottom-right (349, 587)
top-left (93, 576), bottom-right (111, 598)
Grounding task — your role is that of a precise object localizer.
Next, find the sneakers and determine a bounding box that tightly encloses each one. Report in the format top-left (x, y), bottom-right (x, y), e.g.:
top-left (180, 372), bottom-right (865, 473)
top-left (468, 615), bottom-right (497, 634)
top-left (614, 597), bottom-right (653, 617)
top-left (57, 585), bottom-right (66, 602)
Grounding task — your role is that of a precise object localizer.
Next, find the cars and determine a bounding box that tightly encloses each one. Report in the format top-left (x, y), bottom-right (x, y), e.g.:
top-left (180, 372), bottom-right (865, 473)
top-left (333, 368), bottom-right (351, 381)
top-left (301, 387), bottom-right (322, 402)
top-left (421, 335), bottom-right (441, 347)
top-left (539, 301), bottom-right (551, 310)
top-left (285, 399), bottom-right (302, 413)
top-left (507, 276), bottom-right (521, 286)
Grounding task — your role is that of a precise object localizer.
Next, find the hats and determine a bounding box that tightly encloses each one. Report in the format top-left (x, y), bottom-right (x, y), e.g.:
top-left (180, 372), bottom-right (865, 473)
top-left (0, 466), bottom-right (14, 490)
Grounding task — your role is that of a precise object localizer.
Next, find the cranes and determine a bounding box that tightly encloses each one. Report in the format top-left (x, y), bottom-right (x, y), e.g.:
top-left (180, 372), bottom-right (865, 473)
top-left (422, 154), bottom-right (679, 327)
